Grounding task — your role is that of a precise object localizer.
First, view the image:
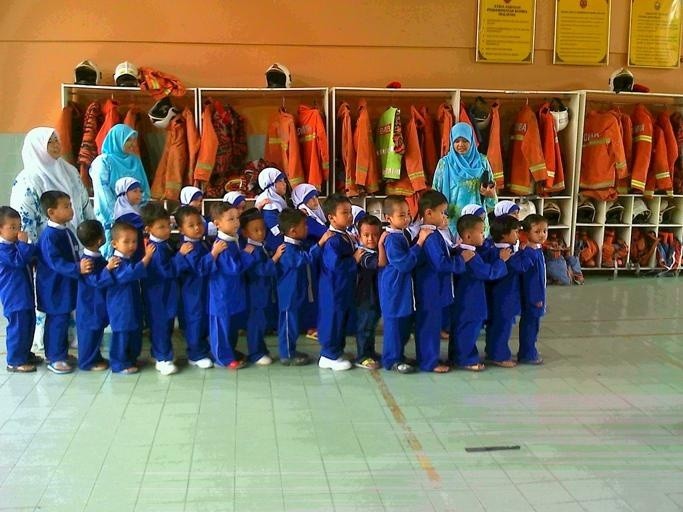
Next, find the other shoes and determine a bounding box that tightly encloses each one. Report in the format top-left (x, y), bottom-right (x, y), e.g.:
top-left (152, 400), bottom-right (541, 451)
top-left (486, 359), bottom-right (517, 367)
top-left (433, 363), bottom-right (450, 372)
top-left (464, 363), bottom-right (484, 370)
top-left (519, 357), bottom-right (543, 365)
top-left (91, 363), bottom-right (108, 371)
top-left (120, 367), bottom-right (139, 374)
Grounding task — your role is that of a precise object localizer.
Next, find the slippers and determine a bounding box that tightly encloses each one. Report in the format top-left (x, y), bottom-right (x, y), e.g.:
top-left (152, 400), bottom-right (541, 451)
top-left (305, 330), bottom-right (319, 341)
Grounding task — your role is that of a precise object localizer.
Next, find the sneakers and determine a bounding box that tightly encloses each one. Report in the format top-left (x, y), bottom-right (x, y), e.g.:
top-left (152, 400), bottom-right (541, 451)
top-left (353, 356), bottom-right (380, 368)
top-left (29, 352), bottom-right (42, 364)
top-left (188, 357), bottom-right (213, 368)
top-left (319, 356), bottom-right (352, 371)
top-left (403, 356), bottom-right (416, 364)
top-left (255, 355), bottom-right (271, 365)
top-left (214, 360), bottom-right (245, 368)
top-left (47, 361), bottom-right (72, 373)
top-left (281, 355), bottom-right (310, 365)
top-left (44, 355), bottom-right (76, 364)
top-left (375, 353), bottom-right (381, 360)
top-left (7, 363), bottom-right (37, 372)
top-left (391, 362), bottom-right (414, 373)
top-left (155, 360), bottom-right (178, 375)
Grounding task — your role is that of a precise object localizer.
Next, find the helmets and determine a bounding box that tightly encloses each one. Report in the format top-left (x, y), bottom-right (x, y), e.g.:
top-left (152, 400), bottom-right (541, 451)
top-left (516, 200), bottom-right (538, 221)
top-left (467, 102), bottom-right (491, 130)
top-left (544, 200), bottom-right (561, 225)
top-left (368, 201), bottom-right (389, 225)
top-left (609, 67), bottom-right (634, 91)
top-left (146, 99), bottom-right (180, 128)
top-left (112, 60), bottom-right (139, 87)
top-left (577, 198), bottom-right (677, 225)
top-left (265, 62), bottom-right (291, 88)
top-left (547, 107), bottom-right (570, 133)
top-left (73, 58), bottom-right (102, 85)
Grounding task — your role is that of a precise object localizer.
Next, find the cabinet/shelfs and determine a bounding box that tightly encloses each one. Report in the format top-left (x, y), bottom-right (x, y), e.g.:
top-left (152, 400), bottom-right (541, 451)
top-left (457, 194), bottom-right (573, 253)
top-left (457, 89), bottom-right (580, 199)
top-left (569, 90), bottom-right (683, 195)
top-left (61, 84), bottom-right (198, 201)
top-left (198, 87), bottom-right (330, 199)
top-left (333, 89), bottom-right (459, 196)
top-left (573, 193), bottom-right (683, 278)
top-left (201, 196), bottom-right (329, 218)
top-left (332, 193), bottom-right (458, 221)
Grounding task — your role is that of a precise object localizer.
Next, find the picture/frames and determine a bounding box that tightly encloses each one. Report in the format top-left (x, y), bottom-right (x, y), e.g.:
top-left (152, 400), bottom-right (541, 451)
top-left (474, 0), bottom-right (537, 65)
top-left (625, 0), bottom-right (683, 70)
top-left (551, 0), bottom-right (613, 67)
